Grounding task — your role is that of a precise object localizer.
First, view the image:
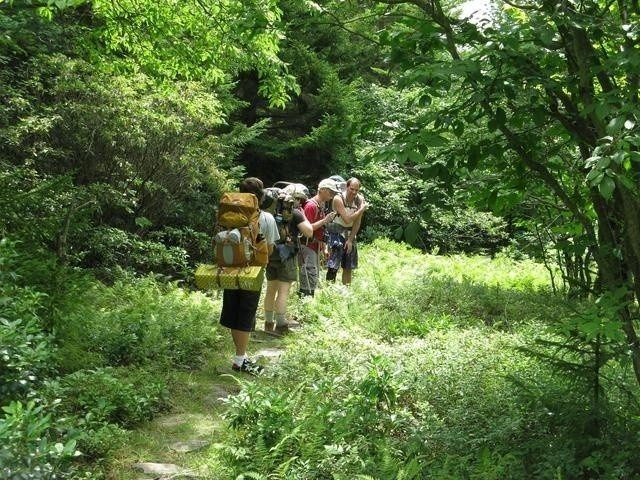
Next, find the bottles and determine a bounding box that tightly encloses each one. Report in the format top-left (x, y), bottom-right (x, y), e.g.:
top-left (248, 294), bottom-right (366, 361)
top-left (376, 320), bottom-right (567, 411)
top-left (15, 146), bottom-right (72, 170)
top-left (256, 232), bottom-right (265, 244)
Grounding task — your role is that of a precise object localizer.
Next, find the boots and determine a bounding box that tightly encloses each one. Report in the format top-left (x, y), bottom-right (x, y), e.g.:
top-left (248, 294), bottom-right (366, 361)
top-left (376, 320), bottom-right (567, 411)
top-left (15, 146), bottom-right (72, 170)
top-left (265, 321), bottom-right (289, 334)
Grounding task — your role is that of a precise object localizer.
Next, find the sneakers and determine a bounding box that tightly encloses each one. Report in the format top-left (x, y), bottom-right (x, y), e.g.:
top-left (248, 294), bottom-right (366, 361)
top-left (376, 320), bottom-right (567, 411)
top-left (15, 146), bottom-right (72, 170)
top-left (232, 356), bottom-right (264, 377)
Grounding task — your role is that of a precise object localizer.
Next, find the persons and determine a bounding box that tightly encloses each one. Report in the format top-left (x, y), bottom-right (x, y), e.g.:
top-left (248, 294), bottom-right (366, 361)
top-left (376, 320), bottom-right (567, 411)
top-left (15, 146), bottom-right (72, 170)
top-left (324, 177), bottom-right (373, 286)
top-left (297, 179), bottom-right (342, 297)
top-left (220, 176), bottom-right (280, 376)
top-left (264, 184), bottom-right (313, 336)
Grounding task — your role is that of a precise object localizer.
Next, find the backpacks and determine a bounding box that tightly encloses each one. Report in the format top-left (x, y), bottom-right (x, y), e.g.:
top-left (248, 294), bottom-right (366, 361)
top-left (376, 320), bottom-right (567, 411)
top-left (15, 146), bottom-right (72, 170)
top-left (212, 192), bottom-right (268, 266)
top-left (324, 175), bottom-right (362, 218)
top-left (259, 187), bottom-right (295, 268)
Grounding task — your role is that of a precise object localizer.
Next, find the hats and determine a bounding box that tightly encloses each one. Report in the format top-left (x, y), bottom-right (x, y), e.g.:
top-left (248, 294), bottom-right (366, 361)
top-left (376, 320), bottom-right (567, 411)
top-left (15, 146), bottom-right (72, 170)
top-left (317, 178), bottom-right (342, 193)
